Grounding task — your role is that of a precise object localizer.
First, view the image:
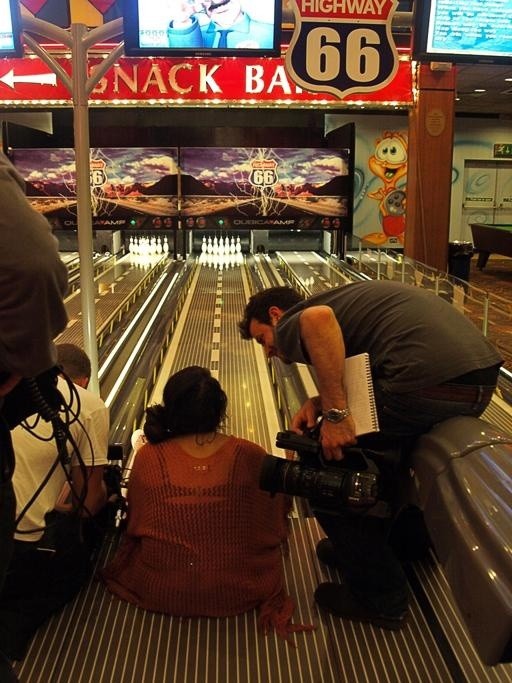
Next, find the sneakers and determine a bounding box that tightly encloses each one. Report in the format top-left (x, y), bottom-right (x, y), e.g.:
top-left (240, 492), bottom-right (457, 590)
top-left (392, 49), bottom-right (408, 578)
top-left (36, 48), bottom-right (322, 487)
top-left (315, 582), bottom-right (405, 632)
top-left (316, 537), bottom-right (338, 566)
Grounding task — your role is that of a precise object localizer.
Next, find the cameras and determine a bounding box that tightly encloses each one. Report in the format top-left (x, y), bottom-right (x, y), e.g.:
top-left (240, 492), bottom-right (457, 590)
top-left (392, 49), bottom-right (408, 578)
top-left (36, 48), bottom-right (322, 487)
top-left (257, 432), bottom-right (381, 508)
top-left (73, 444), bottom-right (125, 549)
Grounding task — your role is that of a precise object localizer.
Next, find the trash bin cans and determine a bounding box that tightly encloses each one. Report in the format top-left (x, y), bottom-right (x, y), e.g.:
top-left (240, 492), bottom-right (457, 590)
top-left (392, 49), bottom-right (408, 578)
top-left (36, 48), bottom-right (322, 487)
top-left (448, 242), bottom-right (473, 293)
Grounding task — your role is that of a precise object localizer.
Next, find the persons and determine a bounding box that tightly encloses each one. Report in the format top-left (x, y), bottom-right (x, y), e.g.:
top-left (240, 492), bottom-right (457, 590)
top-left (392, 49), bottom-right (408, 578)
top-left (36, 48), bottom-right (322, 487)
top-left (238, 279), bottom-right (505, 631)
top-left (94, 365), bottom-right (317, 648)
top-left (1, 144), bottom-right (70, 682)
top-left (9, 341), bottom-right (110, 627)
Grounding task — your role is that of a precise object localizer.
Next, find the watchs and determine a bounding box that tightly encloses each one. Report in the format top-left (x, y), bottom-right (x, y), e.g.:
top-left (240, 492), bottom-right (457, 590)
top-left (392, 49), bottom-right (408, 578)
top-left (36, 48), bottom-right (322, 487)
top-left (322, 408), bottom-right (351, 422)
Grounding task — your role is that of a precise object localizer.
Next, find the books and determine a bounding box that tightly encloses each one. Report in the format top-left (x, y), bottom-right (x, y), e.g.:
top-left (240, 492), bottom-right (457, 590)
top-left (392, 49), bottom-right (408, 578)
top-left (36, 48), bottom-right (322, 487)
top-left (341, 350), bottom-right (381, 437)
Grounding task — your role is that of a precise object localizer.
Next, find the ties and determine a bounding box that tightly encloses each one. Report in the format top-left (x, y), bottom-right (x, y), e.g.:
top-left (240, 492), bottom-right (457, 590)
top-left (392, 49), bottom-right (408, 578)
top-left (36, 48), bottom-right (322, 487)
top-left (217, 30), bottom-right (234, 48)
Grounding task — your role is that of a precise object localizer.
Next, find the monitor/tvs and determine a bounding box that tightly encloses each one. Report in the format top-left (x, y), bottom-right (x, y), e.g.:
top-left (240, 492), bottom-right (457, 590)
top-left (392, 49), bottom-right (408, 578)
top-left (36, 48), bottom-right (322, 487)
top-left (410, 0), bottom-right (511, 65)
top-left (122, 0), bottom-right (282, 58)
top-left (1, 0), bottom-right (24, 59)
